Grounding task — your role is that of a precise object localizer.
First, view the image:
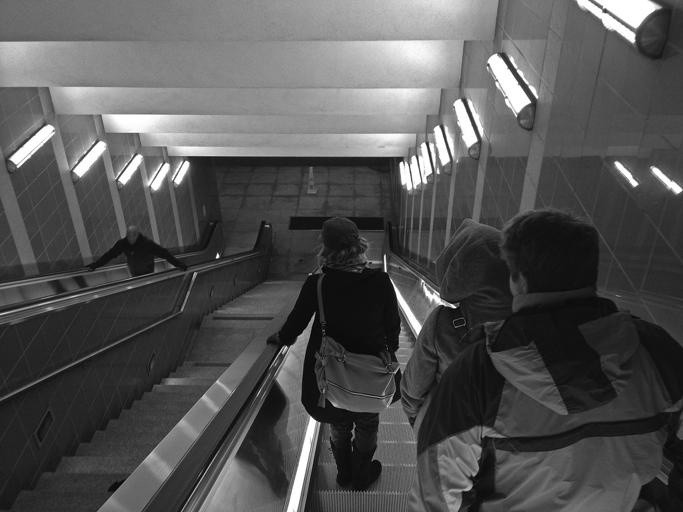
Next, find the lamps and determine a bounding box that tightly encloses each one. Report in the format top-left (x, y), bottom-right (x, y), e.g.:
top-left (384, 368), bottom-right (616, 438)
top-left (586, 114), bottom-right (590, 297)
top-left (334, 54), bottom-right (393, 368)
top-left (171, 160), bottom-right (191, 187)
top-left (72, 140), bottom-right (108, 182)
top-left (6, 121), bottom-right (56, 172)
top-left (577, 0), bottom-right (670, 58)
top-left (148, 162), bottom-right (170, 192)
top-left (398, 98), bottom-right (484, 192)
top-left (116, 153), bottom-right (144, 187)
top-left (487, 52), bottom-right (538, 131)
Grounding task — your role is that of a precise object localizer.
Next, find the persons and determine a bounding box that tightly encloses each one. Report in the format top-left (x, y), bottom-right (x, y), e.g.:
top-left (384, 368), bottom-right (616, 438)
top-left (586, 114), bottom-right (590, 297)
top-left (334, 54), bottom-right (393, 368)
top-left (82, 223), bottom-right (188, 277)
top-left (398, 214), bottom-right (516, 432)
top-left (407, 207), bottom-right (683, 512)
top-left (265, 214), bottom-right (403, 492)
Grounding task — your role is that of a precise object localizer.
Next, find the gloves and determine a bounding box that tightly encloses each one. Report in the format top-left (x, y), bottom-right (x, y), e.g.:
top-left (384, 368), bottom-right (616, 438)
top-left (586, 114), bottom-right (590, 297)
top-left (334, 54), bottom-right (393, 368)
top-left (267, 331), bottom-right (282, 346)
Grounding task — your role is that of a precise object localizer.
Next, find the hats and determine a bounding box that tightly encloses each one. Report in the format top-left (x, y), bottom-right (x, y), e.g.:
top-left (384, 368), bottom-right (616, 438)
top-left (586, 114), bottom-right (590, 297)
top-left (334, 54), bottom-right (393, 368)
top-left (321, 216), bottom-right (360, 249)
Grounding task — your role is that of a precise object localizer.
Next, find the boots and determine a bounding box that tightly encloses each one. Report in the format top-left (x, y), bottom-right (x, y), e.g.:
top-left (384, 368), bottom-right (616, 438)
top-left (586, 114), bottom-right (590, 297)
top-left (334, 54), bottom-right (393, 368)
top-left (352, 438), bottom-right (382, 489)
top-left (329, 436), bottom-right (353, 486)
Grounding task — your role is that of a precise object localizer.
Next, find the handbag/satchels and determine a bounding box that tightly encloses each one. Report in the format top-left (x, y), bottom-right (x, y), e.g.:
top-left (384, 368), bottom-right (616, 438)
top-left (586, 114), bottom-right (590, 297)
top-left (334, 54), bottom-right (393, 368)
top-left (314, 336), bottom-right (400, 413)
